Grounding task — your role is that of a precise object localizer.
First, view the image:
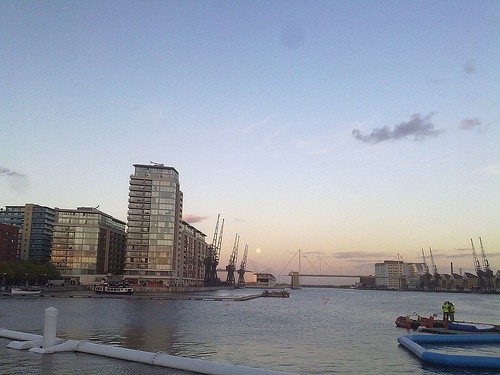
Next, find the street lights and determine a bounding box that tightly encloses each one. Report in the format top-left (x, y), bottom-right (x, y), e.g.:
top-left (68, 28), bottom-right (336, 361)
top-left (44, 274), bottom-right (47, 285)
top-left (25, 273), bottom-right (28, 285)
top-left (3, 273), bottom-right (8, 291)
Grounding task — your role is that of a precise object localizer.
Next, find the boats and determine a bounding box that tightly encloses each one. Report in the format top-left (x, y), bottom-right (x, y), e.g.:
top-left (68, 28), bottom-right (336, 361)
top-left (395, 313), bottom-right (500, 334)
top-left (94, 275), bottom-right (134, 295)
top-left (262, 289), bottom-right (290, 298)
top-left (10, 286), bottom-right (41, 295)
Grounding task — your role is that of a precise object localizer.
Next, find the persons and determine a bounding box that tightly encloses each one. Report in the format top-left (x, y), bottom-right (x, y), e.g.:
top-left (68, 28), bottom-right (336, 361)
top-left (441, 301), bottom-right (455, 321)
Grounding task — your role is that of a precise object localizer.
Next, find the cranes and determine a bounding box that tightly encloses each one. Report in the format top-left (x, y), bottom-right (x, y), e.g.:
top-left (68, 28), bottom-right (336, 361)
top-left (421, 246), bottom-right (441, 291)
top-left (226, 233), bottom-right (249, 287)
top-left (203, 214), bottom-right (225, 288)
top-left (470, 237), bottom-right (497, 293)
